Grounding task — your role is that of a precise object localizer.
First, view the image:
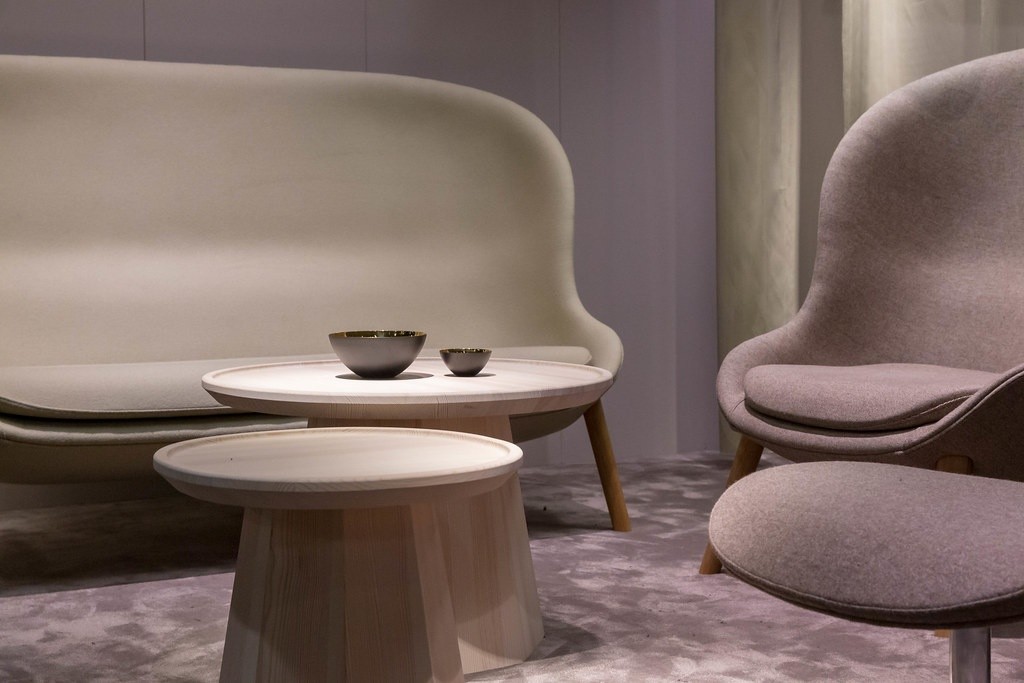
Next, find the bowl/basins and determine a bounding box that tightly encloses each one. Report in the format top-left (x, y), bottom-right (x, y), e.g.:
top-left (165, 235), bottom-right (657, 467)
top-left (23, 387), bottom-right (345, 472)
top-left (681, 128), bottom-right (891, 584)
top-left (439, 348), bottom-right (492, 377)
top-left (328, 330), bottom-right (427, 379)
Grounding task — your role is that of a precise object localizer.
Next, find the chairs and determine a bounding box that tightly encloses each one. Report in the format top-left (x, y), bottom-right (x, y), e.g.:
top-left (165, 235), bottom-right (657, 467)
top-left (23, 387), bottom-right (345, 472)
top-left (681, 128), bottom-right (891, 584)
top-left (697, 48), bottom-right (1024, 576)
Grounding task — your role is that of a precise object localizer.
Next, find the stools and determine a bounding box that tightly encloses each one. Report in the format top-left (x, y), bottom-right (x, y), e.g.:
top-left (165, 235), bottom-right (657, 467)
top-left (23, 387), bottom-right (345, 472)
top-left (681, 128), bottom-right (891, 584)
top-left (707, 460), bottom-right (1024, 683)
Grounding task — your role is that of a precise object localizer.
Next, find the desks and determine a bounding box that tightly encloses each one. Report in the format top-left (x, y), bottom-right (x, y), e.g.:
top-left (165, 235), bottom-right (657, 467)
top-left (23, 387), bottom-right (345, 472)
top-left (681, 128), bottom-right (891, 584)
top-left (152, 426), bottom-right (524, 683)
top-left (203, 358), bottom-right (613, 673)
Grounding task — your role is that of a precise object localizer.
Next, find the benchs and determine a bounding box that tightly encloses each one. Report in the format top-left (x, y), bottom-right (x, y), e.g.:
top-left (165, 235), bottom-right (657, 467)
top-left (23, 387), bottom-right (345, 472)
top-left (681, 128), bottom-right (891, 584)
top-left (0, 54), bottom-right (631, 531)
